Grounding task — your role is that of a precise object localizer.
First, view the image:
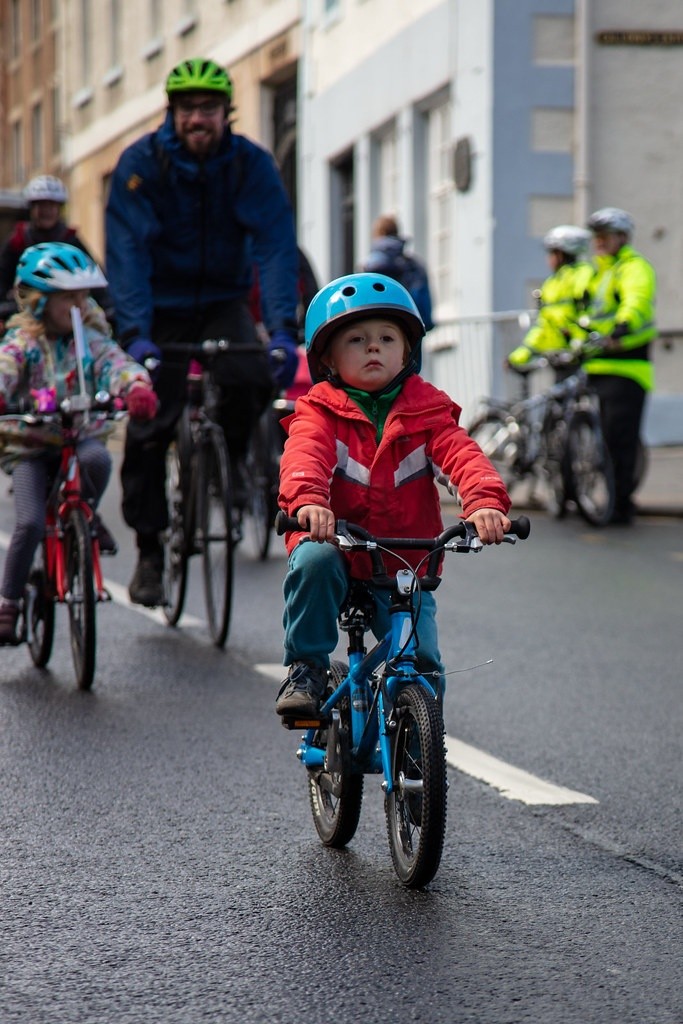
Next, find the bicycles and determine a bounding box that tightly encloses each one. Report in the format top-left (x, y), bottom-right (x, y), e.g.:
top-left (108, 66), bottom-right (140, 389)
top-left (0, 389), bottom-right (163, 692)
top-left (461, 317), bottom-right (650, 532)
top-left (137, 336), bottom-right (298, 651)
top-left (273, 509), bottom-right (531, 891)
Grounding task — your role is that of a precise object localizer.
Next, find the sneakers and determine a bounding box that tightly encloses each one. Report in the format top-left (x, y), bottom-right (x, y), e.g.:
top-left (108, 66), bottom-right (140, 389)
top-left (229, 463), bottom-right (250, 506)
top-left (405, 792), bottom-right (423, 825)
top-left (130, 539), bottom-right (166, 604)
top-left (276, 661), bottom-right (328, 715)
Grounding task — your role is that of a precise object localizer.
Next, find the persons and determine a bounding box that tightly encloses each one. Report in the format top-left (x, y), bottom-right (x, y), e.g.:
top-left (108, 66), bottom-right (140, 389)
top-left (252, 249), bottom-right (319, 346)
top-left (0, 243), bottom-right (156, 645)
top-left (361, 215), bottom-right (435, 330)
top-left (505, 208), bottom-right (656, 529)
top-left (106, 60), bottom-right (301, 604)
top-left (0, 174), bottom-right (106, 322)
top-left (276, 271), bottom-right (512, 828)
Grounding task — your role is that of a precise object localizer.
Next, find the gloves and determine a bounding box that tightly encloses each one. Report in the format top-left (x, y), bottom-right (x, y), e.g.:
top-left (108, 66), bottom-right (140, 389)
top-left (126, 338), bottom-right (163, 381)
top-left (126, 380), bottom-right (157, 422)
top-left (263, 340), bottom-right (298, 387)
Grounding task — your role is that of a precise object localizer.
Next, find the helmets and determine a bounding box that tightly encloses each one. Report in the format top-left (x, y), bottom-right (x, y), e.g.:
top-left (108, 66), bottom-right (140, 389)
top-left (590, 208), bottom-right (636, 233)
top-left (15, 241), bottom-right (109, 293)
top-left (304, 272), bottom-right (426, 385)
top-left (545, 225), bottom-right (593, 256)
top-left (167, 59), bottom-right (233, 100)
top-left (25, 174), bottom-right (67, 204)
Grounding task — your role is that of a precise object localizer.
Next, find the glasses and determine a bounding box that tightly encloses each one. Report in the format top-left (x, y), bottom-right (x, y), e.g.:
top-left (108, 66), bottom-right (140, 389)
top-left (177, 100), bottom-right (222, 115)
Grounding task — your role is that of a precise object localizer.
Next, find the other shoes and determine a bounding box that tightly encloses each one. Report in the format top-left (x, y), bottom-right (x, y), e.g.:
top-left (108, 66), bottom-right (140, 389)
top-left (608, 503), bottom-right (637, 524)
top-left (92, 515), bottom-right (116, 550)
top-left (0, 603), bottom-right (18, 640)
top-left (531, 479), bottom-right (560, 515)
top-left (509, 482), bottom-right (529, 508)
